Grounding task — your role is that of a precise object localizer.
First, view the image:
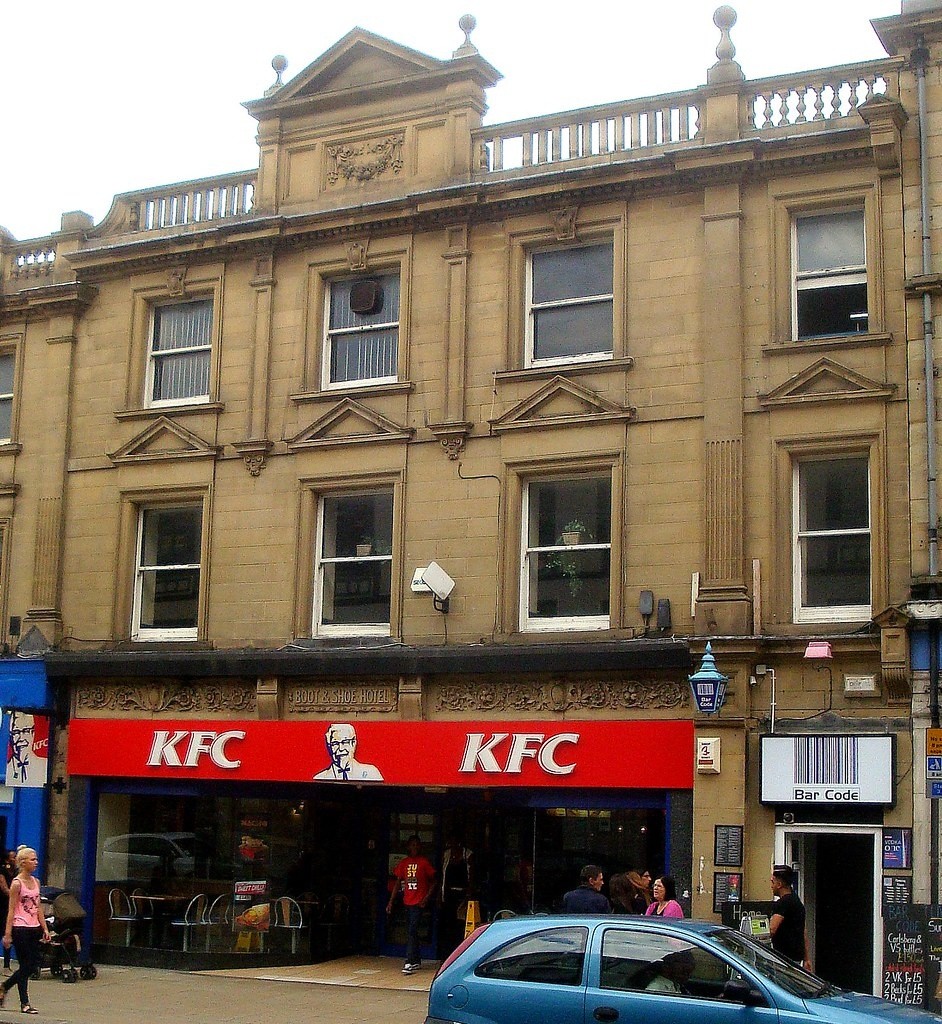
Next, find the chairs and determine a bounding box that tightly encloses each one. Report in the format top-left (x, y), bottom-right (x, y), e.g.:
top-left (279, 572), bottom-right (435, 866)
top-left (108, 887), bottom-right (351, 954)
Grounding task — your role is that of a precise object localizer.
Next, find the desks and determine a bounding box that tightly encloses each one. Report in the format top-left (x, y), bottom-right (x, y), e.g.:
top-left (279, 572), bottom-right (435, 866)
top-left (129, 894), bottom-right (188, 950)
top-left (270, 897), bottom-right (320, 953)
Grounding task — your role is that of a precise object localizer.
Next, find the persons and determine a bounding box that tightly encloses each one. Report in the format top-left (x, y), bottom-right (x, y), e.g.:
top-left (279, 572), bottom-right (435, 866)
top-left (759, 865), bottom-right (812, 972)
top-left (0, 845), bottom-right (51, 1013)
top-left (387, 834), bottom-right (476, 973)
top-left (563, 865), bottom-right (684, 919)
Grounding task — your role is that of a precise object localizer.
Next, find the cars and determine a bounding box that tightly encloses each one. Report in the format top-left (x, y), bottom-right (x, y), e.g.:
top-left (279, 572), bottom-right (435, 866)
top-left (102, 831), bottom-right (247, 882)
top-left (423, 912), bottom-right (942, 1024)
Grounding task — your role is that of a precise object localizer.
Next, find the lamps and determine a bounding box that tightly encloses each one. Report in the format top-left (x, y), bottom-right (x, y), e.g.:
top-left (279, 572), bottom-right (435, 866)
top-left (688, 642), bottom-right (731, 715)
top-left (803, 642), bottom-right (834, 659)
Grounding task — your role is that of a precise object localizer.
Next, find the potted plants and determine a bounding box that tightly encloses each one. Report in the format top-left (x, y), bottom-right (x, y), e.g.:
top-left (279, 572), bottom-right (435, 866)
top-left (546, 518), bottom-right (595, 597)
top-left (356, 533), bottom-right (392, 563)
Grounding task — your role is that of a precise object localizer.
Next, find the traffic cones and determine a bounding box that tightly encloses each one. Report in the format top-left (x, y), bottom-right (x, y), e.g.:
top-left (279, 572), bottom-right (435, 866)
top-left (462, 899), bottom-right (483, 941)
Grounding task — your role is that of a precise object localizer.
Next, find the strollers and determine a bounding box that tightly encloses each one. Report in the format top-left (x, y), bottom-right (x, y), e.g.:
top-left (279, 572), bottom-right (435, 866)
top-left (25, 885), bottom-right (98, 985)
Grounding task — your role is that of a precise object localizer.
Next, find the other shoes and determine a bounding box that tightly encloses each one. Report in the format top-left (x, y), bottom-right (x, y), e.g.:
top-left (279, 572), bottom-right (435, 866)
top-left (412, 964), bottom-right (421, 970)
top-left (1, 967), bottom-right (14, 976)
top-left (402, 964), bottom-right (414, 973)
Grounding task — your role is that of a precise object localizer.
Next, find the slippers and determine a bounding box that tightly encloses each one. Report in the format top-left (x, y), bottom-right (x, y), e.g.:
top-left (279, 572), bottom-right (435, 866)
top-left (22, 1008), bottom-right (38, 1014)
top-left (0, 982), bottom-right (4, 1006)
